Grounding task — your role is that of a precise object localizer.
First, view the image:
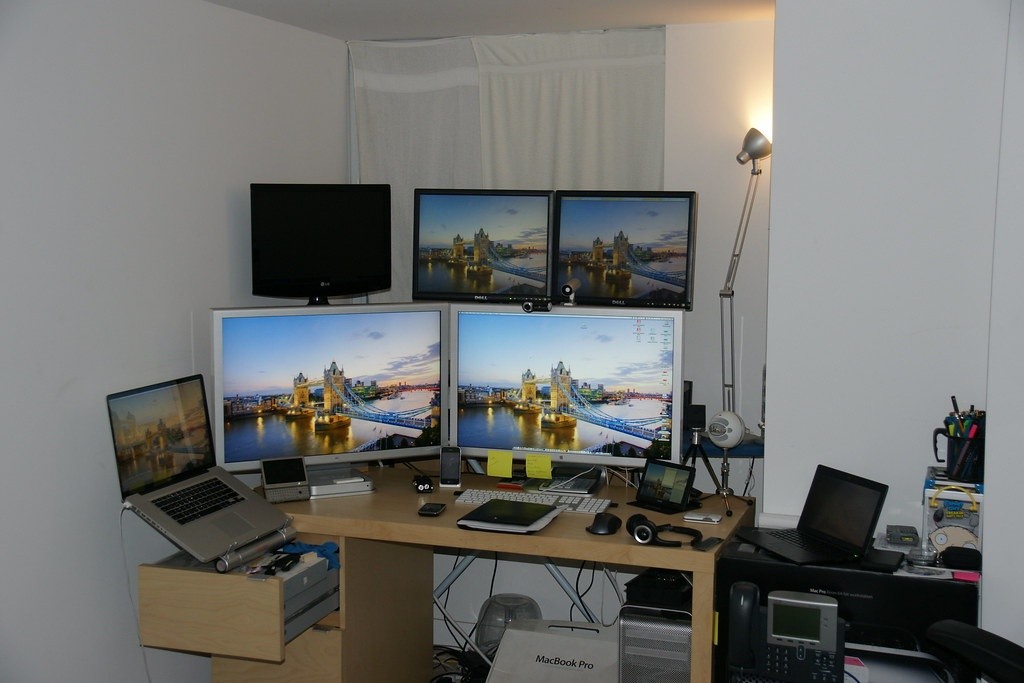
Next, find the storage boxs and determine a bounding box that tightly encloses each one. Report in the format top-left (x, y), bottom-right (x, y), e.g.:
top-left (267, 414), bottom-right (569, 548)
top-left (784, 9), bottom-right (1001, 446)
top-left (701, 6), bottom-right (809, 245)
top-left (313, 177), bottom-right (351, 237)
top-left (484, 620), bottom-right (619, 681)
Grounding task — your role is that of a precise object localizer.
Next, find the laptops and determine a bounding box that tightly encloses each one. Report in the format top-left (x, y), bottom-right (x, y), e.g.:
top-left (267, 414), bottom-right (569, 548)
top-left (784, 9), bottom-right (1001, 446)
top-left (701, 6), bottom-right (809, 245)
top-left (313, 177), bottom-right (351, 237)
top-left (733, 463), bottom-right (890, 566)
top-left (259, 456), bottom-right (312, 504)
top-left (107, 374), bottom-right (290, 564)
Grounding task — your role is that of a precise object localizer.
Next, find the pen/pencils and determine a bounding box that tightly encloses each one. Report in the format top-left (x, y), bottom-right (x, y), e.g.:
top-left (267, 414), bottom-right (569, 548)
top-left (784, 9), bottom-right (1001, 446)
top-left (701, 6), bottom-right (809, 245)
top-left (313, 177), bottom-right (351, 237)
top-left (951, 396), bottom-right (959, 413)
top-left (944, 412), bottom-right (982, 479)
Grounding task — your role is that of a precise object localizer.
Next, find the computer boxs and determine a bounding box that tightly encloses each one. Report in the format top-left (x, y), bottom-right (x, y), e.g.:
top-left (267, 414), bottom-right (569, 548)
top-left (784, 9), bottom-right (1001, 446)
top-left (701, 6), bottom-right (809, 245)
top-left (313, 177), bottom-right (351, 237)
top-left (617, 570), bottom-right (694, 683)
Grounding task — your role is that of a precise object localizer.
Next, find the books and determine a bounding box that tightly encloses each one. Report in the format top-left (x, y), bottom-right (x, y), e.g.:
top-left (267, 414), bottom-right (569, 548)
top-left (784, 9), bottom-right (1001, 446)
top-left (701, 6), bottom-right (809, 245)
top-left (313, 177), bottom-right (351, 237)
top-left (457, 499), bottom-right (558, 535)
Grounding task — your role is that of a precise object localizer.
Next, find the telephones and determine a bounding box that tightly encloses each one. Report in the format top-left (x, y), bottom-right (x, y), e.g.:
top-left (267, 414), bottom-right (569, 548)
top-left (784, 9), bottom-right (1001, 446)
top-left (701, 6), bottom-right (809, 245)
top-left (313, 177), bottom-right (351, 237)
top-left (728, 582), bottom-right (845, 683)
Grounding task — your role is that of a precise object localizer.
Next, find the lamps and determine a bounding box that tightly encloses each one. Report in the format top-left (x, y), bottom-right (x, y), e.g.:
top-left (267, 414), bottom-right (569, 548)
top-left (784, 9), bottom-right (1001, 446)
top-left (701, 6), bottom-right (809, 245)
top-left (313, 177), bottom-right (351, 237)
top-left (702, 128), bottom-right (772, 440)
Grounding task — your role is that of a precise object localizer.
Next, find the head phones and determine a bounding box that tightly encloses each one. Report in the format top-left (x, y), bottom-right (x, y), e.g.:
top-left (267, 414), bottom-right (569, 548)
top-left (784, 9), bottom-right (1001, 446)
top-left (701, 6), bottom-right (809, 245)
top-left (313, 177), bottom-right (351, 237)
top-left (626, 514), bottom-right (702, 547)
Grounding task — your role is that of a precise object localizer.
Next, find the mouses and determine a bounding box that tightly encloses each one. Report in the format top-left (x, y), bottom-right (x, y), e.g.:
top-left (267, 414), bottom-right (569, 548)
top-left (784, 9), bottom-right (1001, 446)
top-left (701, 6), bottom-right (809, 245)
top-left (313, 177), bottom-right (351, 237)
top-left (586, 512), bottom-right (622, 535)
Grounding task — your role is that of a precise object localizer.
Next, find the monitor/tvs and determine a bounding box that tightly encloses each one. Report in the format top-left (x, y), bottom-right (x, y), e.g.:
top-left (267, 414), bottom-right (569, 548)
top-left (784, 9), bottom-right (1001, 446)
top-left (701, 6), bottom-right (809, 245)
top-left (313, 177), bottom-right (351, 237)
top-left (209, 303), bottom-right (451, 500)
top-left (449, 301), bottom-right (685, 495)
top-left (550, 190), bottom-right (698, 312)
top-left (249, 183), bottom-right (392, 305)
top-left (413, 188), bottom-right (555, 304)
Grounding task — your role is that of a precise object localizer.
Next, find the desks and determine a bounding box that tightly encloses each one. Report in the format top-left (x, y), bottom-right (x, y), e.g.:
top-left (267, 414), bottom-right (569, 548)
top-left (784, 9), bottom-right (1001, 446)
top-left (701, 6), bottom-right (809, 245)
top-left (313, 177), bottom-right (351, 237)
top-left (138, 431), bottom-right (764, 683)
top-left (714, 528), bottom-right (978, 683)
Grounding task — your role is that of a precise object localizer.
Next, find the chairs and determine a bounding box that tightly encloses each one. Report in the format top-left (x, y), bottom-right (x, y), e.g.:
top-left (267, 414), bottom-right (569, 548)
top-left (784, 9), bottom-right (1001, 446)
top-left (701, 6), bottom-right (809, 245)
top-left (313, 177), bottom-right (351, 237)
top-left (927, 619), bottom-right (1024, 683)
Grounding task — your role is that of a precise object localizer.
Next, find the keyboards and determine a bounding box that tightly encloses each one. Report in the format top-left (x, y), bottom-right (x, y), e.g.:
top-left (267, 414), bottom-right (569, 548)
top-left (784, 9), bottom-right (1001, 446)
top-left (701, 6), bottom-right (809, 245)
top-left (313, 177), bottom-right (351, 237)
top-left (454, 489), bottom-right (613, 514)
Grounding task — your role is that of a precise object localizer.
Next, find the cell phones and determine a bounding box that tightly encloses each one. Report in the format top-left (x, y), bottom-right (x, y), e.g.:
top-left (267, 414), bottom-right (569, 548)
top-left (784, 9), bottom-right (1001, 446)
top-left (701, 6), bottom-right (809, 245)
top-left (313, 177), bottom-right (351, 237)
top-left (439, 446), bottom-right (461, 487)
top-left (418, 503), bottom-right (446, 517)
